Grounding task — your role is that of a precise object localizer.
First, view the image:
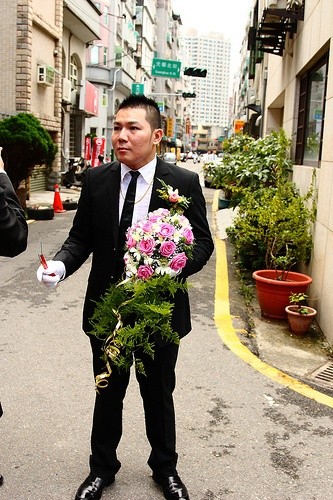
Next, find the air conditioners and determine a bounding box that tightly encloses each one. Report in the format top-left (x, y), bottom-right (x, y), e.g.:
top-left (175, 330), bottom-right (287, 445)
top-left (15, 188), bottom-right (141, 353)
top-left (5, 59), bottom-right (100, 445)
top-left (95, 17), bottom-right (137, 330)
top-left (256, 0), bottom-right (304, 57)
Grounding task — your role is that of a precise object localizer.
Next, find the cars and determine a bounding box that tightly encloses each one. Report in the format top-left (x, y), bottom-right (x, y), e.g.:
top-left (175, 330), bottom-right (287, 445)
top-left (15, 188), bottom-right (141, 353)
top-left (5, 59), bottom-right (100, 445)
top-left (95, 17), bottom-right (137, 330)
top-left (180, 153), bottom-right (188, 162)
top-left (162, 152), bottom-right (176, 165)
top-left (188, 153), bottom-right (193, 159)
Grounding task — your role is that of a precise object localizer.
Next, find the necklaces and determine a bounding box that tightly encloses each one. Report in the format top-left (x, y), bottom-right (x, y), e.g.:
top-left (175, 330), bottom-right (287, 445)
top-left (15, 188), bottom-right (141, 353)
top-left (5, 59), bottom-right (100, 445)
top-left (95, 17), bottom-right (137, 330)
top-left (120, 180), bottom-right (152, 204)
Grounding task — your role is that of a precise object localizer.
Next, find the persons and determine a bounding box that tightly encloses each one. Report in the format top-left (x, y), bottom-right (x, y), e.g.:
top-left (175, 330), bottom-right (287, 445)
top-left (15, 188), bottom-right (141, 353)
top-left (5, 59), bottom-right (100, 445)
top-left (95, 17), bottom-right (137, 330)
top-left (36, 93), bottom-right (214, 500)
top-left (192, 151), bottom-right (214, 164)
top-left (0, 146), bottom-right (28, 484)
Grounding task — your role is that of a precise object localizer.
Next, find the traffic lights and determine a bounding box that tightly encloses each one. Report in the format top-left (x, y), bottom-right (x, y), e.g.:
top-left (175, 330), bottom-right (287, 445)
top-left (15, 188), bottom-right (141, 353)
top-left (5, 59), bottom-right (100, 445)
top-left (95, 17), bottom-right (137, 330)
top-left (182, 92), bottom-right (196, 98)
top-left (184, 67), bottom-right (207, 78)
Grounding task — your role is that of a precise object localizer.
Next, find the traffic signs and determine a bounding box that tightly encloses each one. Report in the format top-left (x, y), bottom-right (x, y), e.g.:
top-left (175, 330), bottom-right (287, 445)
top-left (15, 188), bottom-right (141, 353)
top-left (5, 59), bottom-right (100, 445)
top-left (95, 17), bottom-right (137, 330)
top-left (151, 58), bottom-right (182, 78)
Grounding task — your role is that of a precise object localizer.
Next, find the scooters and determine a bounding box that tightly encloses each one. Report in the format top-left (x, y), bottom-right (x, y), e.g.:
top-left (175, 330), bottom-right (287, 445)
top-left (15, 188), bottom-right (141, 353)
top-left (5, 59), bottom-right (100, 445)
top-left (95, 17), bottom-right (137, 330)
top-left (60, 155), bottom-right (89, 189)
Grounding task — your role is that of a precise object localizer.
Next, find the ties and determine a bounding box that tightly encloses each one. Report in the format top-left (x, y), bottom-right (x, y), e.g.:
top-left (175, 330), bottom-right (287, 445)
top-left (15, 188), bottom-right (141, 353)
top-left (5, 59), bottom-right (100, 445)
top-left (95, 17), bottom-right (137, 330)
top-left (119, 170), bottom-right (139, 236)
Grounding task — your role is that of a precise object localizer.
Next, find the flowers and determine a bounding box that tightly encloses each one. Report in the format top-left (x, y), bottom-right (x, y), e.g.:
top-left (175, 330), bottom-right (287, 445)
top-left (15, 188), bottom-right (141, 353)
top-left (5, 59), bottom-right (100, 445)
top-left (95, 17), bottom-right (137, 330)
top-left (88, 177), bottom-right (197, 392)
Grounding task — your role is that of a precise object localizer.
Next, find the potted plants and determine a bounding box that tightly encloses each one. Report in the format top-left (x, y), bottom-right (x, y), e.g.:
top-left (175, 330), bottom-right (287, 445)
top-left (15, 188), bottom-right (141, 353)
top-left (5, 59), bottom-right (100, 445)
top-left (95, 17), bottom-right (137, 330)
top-left (218, 185), bottom-right (233, 209)
top-left (251, 169), bottom-right (319, 320)
top-left (202, 162), bottom-right (215, 187)
top-left (285, 292), bottom-right (317, 334)
top-left (0, 112), bottom-right (59, 210)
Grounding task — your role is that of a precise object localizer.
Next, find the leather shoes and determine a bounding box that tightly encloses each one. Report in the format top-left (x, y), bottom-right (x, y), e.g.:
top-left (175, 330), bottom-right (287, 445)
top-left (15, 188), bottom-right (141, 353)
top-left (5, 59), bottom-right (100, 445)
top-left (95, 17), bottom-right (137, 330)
top-left (153, 472), bottom-right (189, 500)
top-left (74, 474), bottom-right (105, 500)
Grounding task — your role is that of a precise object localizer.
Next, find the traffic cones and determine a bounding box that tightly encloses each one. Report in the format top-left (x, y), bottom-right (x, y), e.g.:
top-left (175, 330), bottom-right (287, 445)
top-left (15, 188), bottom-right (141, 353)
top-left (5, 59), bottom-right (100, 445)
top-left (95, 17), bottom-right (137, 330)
top-left (53, 184), bottom-right (66, 213)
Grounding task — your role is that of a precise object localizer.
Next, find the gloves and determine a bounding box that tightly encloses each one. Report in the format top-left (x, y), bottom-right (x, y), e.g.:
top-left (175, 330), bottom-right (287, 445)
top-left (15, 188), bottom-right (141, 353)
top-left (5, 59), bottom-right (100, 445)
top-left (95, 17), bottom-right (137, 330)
top-left (36, 260), bottom-right (65, 289)
